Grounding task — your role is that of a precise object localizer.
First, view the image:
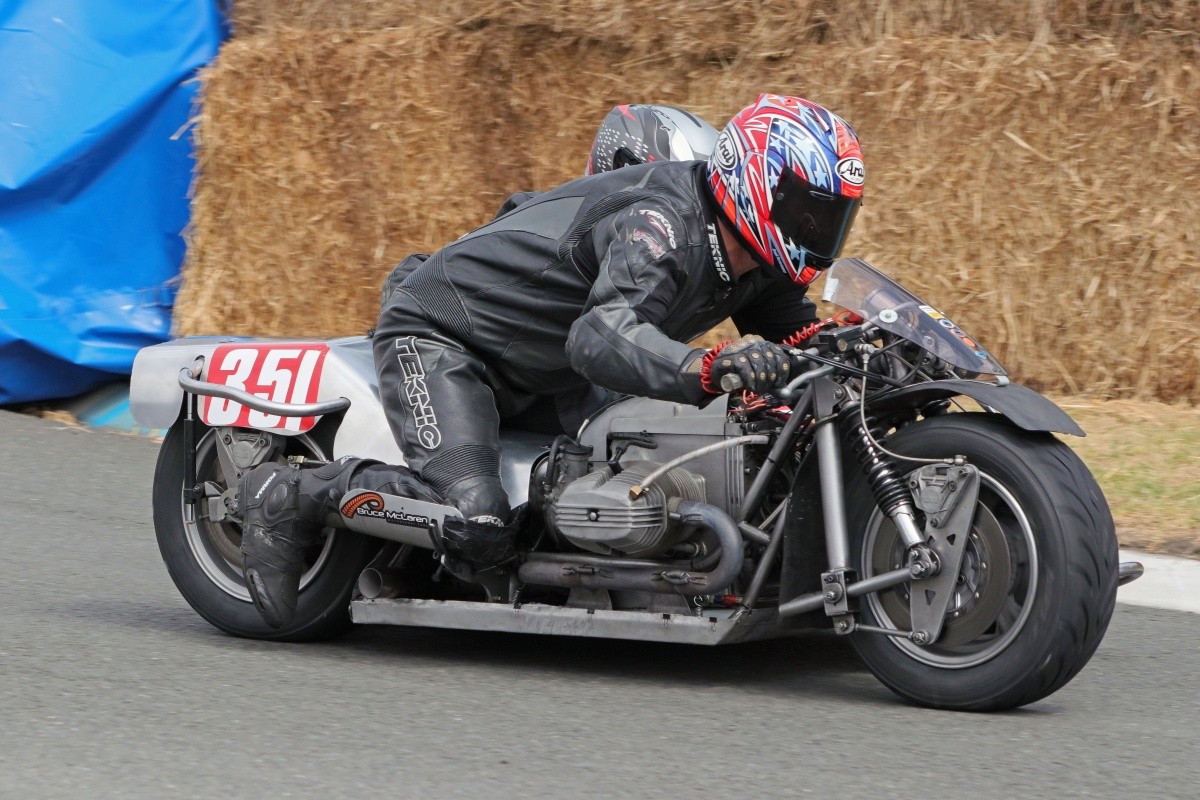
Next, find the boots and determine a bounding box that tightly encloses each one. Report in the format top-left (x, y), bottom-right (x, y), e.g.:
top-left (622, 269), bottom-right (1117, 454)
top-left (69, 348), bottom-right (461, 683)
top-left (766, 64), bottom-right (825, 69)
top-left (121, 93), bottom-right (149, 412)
top-left (240, 458), bottom-right (384, 630)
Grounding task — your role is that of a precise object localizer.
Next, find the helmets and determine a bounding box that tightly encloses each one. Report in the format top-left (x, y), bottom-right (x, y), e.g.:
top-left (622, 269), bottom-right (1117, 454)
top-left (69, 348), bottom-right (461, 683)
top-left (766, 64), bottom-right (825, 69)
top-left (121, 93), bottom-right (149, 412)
top-left (706, 93), bottom-right (865, 287)
top-left (585, 103), bottom-right (722, 177)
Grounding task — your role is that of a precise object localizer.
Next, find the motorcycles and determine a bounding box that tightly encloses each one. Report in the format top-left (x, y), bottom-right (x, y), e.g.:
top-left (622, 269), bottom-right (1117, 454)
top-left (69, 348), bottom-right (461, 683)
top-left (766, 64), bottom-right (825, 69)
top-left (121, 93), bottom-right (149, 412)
top-left (126, 255), bottom-right (1145, 714)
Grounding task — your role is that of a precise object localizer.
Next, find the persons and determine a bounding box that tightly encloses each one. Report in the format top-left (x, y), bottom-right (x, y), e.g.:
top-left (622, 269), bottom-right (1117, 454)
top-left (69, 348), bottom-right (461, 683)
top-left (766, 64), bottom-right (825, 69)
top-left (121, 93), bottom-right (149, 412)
top-left (238, 93), bottom-right (971, 630)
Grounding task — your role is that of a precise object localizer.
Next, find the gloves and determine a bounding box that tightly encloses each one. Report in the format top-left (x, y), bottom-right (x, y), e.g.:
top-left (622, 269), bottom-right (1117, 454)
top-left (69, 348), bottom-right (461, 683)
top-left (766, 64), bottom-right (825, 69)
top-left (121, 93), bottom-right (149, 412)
top-left (678, 333), bottom-right (791, 410)
